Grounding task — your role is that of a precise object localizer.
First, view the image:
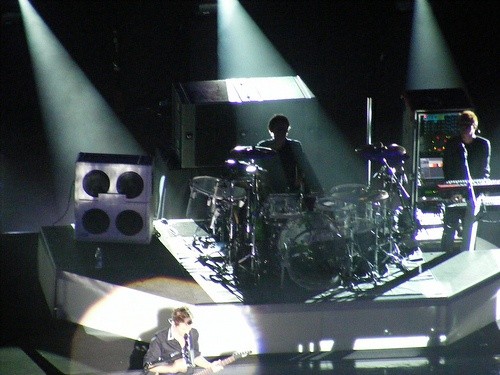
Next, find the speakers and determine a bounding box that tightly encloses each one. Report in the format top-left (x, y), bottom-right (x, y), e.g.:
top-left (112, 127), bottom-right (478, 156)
top-left (75, 151), bottom-right (156, 245)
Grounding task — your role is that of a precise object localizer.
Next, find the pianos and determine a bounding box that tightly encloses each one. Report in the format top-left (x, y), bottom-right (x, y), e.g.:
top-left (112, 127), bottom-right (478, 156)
top-left (435, 178), bottom-right (500, 250)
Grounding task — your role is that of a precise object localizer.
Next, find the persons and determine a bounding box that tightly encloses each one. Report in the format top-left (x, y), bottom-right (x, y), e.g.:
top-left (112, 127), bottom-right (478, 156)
top-left (257, 115), bottom-right (312, 194)
top-left (143, 306), bottom-right (224, 375)
top-left (440, 111), bottom-right (490, 251)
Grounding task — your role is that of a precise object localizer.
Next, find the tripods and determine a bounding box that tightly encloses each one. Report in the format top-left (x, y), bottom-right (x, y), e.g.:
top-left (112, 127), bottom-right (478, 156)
top-left (207, 171), bottom-right (422, 286)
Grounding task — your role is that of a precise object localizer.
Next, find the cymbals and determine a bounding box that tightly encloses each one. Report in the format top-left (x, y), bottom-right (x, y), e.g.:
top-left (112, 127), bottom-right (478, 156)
top-left (219, 144), bottom-right (277, 181)
top-left (330, 188), bottom-right (389, 201)
top-left (356, 143), bottom-right (406, 157)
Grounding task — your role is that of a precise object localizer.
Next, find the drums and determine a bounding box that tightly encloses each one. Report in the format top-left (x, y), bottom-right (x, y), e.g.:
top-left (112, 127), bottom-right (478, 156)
top-left (268, 192), bottom-right (306, 217)
top-left (278, 211), bottom-right (350, 290)
top-left (328, 182), bottom-right (375, 234)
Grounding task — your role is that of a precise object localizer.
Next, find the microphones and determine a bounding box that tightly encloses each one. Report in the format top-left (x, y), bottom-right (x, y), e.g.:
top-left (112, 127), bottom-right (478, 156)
top-left (183, 333), bottom-right (189, 348)
